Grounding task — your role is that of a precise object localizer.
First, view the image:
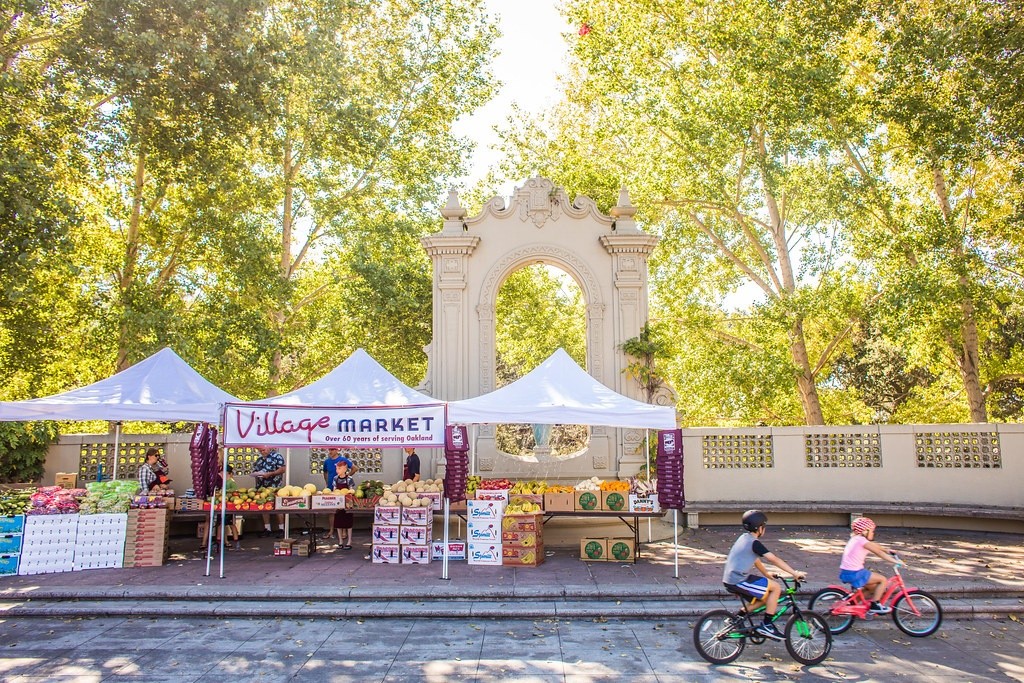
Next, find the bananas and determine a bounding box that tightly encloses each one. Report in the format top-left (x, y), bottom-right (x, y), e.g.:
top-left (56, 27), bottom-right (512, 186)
top-left (505, 479), bottom-right (548, 514)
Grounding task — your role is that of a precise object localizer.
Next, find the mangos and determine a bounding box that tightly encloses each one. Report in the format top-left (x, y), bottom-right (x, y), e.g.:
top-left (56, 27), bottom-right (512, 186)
top-left (207, 487), bottom-right (280, 504)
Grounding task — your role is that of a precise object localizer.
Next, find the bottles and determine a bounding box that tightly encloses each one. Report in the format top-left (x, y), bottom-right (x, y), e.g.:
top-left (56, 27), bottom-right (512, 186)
top-left (158, 459), bottom-right (168, 467)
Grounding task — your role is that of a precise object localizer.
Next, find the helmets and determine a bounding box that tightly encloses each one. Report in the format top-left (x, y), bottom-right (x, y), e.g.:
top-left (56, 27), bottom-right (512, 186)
top-left (741, 510), bottom-right (767, 531)
top-left (850, 518), bottom-right (877, 535)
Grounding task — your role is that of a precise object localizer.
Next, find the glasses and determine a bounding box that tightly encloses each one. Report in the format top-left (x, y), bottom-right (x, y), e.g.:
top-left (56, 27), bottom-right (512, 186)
top-left (328, 449), bottom-right (337, 451)
top-left (154, 454), bottom-right (162, 458)
top-left (165, 482), bottom-right (171, 484)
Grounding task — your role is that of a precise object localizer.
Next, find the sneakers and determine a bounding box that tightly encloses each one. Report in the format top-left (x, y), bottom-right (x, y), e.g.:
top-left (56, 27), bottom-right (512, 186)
top-left (869, 601), bottom-right (892, 614)
top-left (724, 618), bottom-right (740, 631)
top-left (755, 621), bottom-right (788, 641)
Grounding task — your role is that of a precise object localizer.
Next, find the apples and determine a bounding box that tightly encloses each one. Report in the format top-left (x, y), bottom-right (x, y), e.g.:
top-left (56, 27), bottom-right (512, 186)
top-left (480, 478), bottom-right (515, 500)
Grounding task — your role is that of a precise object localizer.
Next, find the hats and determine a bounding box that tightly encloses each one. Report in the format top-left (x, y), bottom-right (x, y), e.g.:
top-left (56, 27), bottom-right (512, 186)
top-left (160, 476), bottom-right (173, 483)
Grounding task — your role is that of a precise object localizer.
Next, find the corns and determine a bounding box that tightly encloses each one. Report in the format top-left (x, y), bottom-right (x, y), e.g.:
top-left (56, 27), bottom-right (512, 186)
top-left (0, 487), bottom-right (38, 516)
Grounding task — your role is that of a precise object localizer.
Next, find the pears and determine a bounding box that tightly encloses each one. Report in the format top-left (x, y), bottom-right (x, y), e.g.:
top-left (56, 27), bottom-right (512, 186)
top-left (467, 475), bottom-right (480, 494)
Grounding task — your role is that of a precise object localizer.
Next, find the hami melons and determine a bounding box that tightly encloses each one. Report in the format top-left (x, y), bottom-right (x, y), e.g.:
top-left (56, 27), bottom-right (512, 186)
top-left (277, 483), bottom-right (317, 496)
top-left (379, 479), bottom-right (444, 506)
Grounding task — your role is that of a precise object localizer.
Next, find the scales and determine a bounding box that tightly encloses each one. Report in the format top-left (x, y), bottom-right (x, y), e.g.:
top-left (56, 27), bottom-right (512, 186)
top-left (185, 489), bottom-right (195, 496)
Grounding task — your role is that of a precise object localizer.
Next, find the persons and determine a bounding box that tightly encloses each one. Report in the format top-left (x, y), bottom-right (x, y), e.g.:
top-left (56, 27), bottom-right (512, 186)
top-left (403, 448), bottom-right (420, 482)
top-left (139, 448), bottom-right (171, 494)
top-left (253, 448), bottom-right (286, 539)
top-left (723, 510), bottom-right (807, 641)
top-left (839, 518), bottom-right (904, 616)
top-left (322, 448), bottom-right (358, 539)
top-left (332, 461), bottom-right (355, 550)
top-left (200, 464), bottom-right (241, 552)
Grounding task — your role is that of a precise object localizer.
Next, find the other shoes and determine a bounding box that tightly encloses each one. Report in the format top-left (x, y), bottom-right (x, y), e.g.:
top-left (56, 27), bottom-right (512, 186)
top-left (276, 529), bottom-right (284, 538)
top-left (322, 534), bottom-right (334, 539)
top-left (338, 534), bottom-right (348, 540)
top-left (336, 543), bottom-right (343, 549)
top-left (343, 544), bottom-right (353, 550)
top-left (258, 528), bottom-right (273, 538)
top-left (192, 541), bottom-right (242, 555)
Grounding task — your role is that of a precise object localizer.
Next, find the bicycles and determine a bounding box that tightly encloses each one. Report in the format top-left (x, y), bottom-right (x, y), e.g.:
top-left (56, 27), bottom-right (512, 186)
top-left (693, 574), bottom-right (832, 668)
top-left (809, 550), bottom-right (944, 638)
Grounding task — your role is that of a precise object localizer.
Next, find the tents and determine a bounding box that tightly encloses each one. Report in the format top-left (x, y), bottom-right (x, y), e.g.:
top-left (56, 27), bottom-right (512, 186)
top-left (446, 348), bottom-right (678, 577)
top-left (0, 347), bottom-right (244, 575)
top-left (220, 347), bottom-right (446, 579)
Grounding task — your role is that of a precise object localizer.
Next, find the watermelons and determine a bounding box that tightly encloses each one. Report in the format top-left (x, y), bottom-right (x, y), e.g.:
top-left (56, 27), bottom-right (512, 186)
top-left (355, 480), bottom-right (384, 499)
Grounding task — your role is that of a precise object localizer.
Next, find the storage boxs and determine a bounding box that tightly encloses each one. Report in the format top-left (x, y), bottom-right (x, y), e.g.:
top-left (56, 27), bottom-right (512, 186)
top-left (311, 493), bottom-right (345, 509)
top-left (467, 489), bottom-right (661, 568)
top-left (203, 501), bottom-right (274, 511)
top-left (274, 495), bottom-right (312, 509)
top-left (274, 539), bottom-right (314, 556)
top-left (345, 490), bottom-right (474, 565)
top-left (175, 497), bottom-right (205, 510)
top-left (197, 521), bottom-right (206, 537)
top-left (0, 495), bottom-right (174, 578)
top-left (55, 472), bottom-right (78, 489)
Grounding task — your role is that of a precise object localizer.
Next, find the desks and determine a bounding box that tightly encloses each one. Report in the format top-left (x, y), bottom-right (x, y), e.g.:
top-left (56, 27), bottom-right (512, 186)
top-left (543, 510), bottom-right (670, 563)
top-left (345, 508), bottom-right (467, 559)
top-left (169, 504), bottom-right (341, 559)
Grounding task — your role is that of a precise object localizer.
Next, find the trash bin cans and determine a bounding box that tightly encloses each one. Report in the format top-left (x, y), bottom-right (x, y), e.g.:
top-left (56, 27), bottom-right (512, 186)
top-left (227, 516), bottom-right (244, 541)
